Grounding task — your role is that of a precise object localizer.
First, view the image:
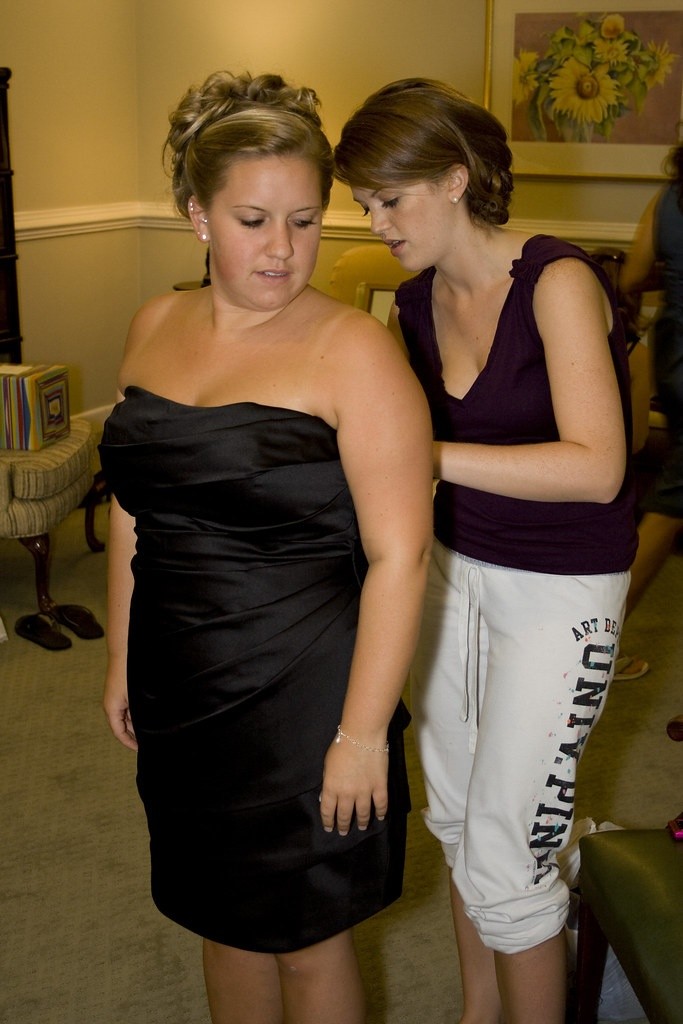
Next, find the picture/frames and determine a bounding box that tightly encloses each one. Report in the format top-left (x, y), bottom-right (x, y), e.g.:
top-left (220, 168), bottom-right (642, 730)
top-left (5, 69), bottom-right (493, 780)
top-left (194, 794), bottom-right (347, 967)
top-left (480, 1), bottom-right (683, 180)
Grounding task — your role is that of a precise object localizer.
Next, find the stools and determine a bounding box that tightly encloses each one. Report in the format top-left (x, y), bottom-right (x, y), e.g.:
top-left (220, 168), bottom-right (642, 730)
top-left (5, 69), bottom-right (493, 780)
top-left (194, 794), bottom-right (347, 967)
top-left (0, 419), bottom-right (105, 621)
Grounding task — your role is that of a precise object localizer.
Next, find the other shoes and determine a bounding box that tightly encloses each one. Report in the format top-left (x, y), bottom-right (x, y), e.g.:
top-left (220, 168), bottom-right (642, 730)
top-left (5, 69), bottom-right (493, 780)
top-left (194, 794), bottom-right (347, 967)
top-left (614, 655), bottom-right (648, 681)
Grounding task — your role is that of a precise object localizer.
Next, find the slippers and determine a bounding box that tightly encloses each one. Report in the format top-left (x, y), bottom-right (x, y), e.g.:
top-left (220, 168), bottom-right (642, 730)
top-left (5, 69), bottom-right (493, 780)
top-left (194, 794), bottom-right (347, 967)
top-left (15, 613), bottom-right (72, 651)
top-left (51, 604), bottom-right (105, 640)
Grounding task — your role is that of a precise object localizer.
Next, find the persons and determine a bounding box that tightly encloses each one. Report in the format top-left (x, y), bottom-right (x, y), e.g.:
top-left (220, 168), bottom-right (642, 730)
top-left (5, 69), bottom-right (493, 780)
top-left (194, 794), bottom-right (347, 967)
top-left (329, 77), bottom-right (633, 1024)
top-left (106, 71), bottom-right (435, 1024)
top-left (614, 138), bottom-right (683, 682)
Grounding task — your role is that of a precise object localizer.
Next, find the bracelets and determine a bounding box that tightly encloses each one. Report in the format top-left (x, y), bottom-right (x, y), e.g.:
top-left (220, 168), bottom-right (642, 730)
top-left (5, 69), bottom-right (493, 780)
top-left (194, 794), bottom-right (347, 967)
top-left (336, 727), bottom-right (391, 753)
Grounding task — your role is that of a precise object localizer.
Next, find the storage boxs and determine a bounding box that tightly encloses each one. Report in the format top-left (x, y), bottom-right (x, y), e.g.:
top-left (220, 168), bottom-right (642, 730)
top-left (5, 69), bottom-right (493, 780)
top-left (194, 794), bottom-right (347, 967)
top-left (0, 362), bottom-right (73, 451)
